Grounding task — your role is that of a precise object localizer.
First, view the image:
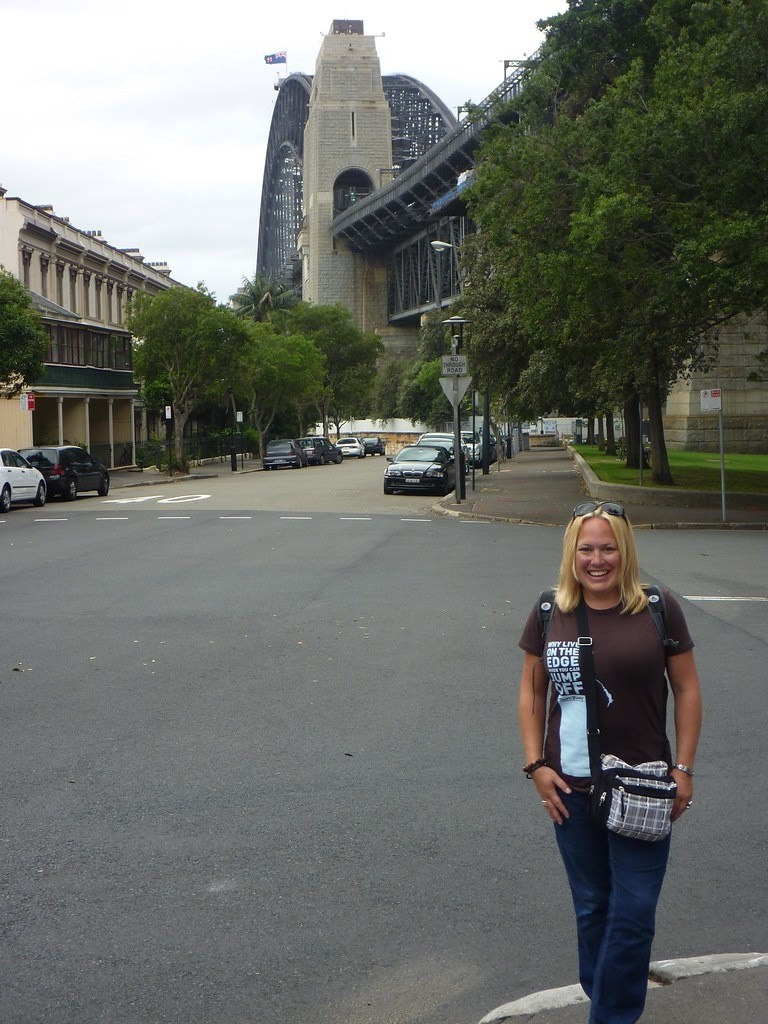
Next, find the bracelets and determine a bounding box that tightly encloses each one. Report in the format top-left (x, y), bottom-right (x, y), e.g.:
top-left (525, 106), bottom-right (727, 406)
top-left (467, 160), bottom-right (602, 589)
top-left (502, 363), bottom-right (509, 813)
top-left (673, 763), bottom-right (694, 776)
top-left (523, 759), bottom-right (545, 780)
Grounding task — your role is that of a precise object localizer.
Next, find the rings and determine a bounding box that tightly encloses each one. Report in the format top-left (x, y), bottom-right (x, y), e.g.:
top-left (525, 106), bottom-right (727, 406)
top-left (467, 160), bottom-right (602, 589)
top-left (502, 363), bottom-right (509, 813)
top-left (542, 801), bottom-right (547, 803)
top-left (687, 801), bottom-right (692, 809)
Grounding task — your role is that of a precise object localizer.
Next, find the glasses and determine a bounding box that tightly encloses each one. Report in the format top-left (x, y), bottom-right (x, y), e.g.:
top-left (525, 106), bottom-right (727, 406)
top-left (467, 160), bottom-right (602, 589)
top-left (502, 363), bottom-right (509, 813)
top-left (573, 501), bottom-right (628, 527)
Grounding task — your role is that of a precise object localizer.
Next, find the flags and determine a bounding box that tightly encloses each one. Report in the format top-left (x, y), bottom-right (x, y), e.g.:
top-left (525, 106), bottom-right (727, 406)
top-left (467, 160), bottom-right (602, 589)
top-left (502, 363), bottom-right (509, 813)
top-left (264, 51), bottom-right (286, 64)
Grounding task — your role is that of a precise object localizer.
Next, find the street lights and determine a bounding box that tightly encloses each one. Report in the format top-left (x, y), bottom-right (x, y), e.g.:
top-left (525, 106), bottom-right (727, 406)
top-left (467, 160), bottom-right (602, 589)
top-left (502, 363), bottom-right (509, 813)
top-left (165, 403), bottom-right (172, 477)
top-left (236, 411), bottom-right (244, 471)
top-left (439, 315), bottom-right (474, 506)
top-left (430, 239), bottom-right (478, 492)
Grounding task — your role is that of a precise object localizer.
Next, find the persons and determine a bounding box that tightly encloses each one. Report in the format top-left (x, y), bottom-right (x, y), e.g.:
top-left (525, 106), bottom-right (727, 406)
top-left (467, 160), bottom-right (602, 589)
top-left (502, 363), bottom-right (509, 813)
top-left (518, 500), bottom-right (703, 1024)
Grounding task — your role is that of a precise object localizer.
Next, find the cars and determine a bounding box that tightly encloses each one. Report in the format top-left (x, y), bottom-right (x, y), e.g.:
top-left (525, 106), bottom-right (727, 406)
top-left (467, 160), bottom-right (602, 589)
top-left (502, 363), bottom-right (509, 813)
top-left (295, 436), bottom-right (344, 466)
top-left (262, 439), bottom-right (309, 472)
top-left (18, 445), bottom-right (110, 501)
top-left (0, 448), bottom-right (48, 514)
top-left (383, 444), bottom-right (455, 496)
top-left (335, 437), bottom-right (366, 459)
top-left (415, 430), bottom-right (507, 475)
top-left (359, 436), bottom-right (385, 457)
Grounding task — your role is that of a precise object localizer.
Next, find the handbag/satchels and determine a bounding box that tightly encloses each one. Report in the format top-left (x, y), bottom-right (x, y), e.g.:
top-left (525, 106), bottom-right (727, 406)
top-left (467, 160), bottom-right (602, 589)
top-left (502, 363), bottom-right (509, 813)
top-left (591, 755), bottom-right (677, 842)
